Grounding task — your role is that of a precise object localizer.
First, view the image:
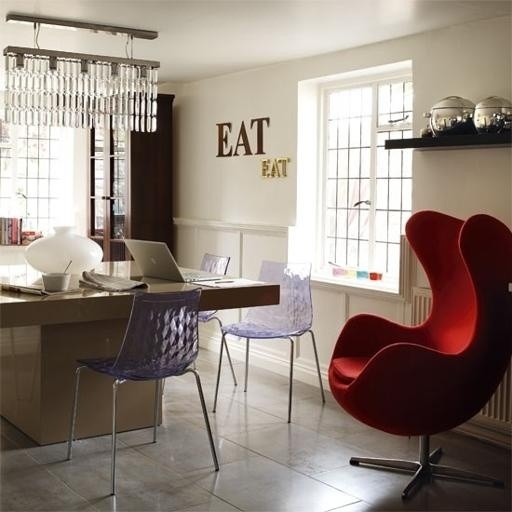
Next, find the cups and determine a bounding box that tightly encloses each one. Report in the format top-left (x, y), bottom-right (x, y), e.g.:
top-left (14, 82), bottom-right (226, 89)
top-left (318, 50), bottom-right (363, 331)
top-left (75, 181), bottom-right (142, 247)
top-left (41, 272), bottom-right (71, 293)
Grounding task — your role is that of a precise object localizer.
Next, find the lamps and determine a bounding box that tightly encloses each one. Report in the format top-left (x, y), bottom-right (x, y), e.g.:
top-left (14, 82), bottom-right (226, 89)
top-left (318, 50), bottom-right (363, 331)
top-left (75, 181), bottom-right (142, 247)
top-left (2, 13), bottom-right (160, 135)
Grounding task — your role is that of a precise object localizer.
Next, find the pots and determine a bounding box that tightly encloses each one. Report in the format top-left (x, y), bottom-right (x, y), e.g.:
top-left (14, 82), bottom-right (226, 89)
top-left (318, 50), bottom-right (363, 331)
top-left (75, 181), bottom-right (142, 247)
top-left (425, 96), bottom-right (475, 136)
top-left (472, 95), bottom-right (512, 135)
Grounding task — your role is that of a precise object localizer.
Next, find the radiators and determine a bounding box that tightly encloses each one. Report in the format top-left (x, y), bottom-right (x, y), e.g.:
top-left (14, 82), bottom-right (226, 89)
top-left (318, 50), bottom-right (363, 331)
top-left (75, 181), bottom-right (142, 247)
top-left (407, 284), bottom-right (512, 438)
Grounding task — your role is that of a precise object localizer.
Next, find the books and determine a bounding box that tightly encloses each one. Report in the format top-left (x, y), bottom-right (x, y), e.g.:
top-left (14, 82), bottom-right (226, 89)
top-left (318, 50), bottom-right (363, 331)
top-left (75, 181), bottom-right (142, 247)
top-left (0, 284), bottom-right (84, 296)
top-left (0, 218), bottom-right (45, 247)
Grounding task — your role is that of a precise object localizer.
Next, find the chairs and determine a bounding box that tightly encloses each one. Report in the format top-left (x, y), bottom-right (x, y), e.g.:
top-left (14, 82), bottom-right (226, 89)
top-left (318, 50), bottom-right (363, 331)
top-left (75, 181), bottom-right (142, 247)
top-left (327, 212), bottom-right (511, 500)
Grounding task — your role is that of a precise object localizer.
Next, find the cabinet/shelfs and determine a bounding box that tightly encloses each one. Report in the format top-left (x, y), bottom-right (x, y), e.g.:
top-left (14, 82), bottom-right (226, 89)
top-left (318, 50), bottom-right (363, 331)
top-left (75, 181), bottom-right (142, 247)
top-left (81, 92), bottom-right (176, 264)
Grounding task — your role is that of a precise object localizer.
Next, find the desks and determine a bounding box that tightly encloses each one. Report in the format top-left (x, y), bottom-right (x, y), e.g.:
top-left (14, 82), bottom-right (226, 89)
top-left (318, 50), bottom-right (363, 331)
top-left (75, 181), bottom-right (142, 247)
top-left (0, 260), bottom-right (280, 451)
